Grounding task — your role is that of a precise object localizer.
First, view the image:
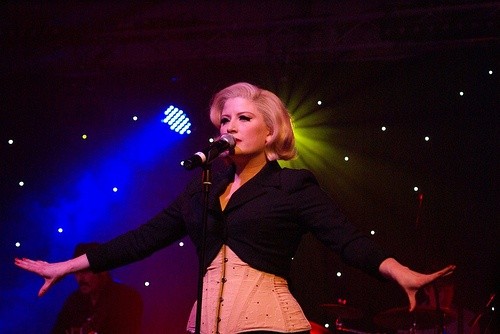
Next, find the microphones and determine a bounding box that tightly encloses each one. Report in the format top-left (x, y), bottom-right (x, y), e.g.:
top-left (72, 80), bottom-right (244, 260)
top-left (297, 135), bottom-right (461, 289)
top-left (181, 133), bottom-right (234, 170)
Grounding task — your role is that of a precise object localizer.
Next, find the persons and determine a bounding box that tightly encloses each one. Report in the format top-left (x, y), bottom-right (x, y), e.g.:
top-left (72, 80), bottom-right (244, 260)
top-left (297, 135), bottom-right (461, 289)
top-left (49, 240), bottom-right (145, 334)
top-left (15, 79), bottom-right (460, 333)
